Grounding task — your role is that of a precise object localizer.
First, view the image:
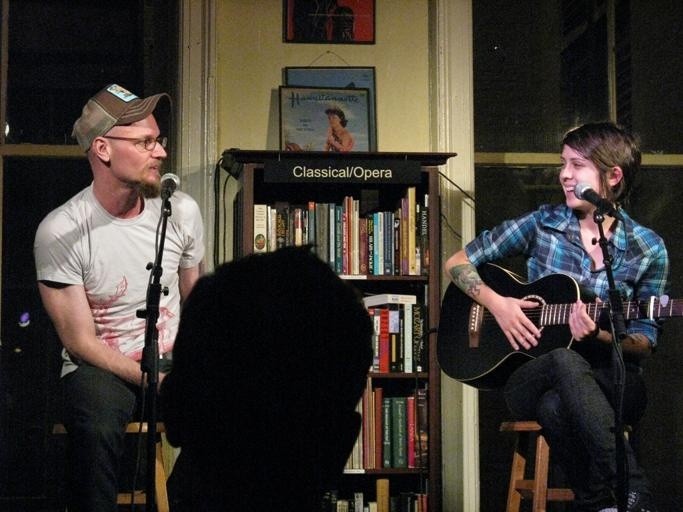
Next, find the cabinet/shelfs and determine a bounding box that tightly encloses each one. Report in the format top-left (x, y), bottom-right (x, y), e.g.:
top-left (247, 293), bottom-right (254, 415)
top-left (218, 143), bottom-right (454, 512)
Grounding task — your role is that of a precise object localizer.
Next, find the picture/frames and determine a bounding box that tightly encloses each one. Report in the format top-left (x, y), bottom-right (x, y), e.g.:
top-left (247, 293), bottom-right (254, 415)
top-left (281, 0), bottom-right (376, 44)
top-left (281, 65), bottom-right (377, 151)
top-left (278, 85), bottom-right (371, 153)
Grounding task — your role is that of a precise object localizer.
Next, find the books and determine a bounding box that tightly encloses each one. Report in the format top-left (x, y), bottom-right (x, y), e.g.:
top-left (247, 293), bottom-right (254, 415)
top-left (335, 294), bottom-right (428, 511)
top-left (250, 184), bottom-right (429, 275)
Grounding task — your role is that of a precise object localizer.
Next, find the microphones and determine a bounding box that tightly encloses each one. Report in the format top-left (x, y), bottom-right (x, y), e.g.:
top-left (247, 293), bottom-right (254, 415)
top-left (573, 183), bottom-right (624, 222)
top-left (160, 173), bottom-right (180, 207)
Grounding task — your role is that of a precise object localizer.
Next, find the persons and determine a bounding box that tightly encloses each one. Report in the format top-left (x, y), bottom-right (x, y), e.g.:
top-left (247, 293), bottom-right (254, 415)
top-left (322, 106), bottom-right (354, 153)
top-left (442, 121), bottom-right (669, 511)
top-left (154, 242), bottom-right (374, 511)
top-left (33, 83), bottom-right (206, 512)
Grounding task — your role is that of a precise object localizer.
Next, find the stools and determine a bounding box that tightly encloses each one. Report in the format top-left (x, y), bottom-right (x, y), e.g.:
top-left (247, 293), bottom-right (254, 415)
top-left (497, 414), bottom-right (635, 511)
top-left (49, 411), bottom-right (174, 511)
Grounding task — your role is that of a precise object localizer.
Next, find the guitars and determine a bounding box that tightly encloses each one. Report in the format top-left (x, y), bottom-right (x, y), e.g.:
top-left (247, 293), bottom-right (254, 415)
top-left (437, 263), bottom-right (683, 391)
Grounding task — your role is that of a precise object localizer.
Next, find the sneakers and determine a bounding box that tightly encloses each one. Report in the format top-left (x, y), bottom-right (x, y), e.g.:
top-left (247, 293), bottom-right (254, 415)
top-left (622, 487), bottom-right (657, 512)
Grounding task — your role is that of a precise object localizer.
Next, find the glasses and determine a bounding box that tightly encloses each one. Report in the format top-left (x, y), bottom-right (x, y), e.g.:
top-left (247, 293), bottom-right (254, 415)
top-left (103, 136), bottom-right (168, 152)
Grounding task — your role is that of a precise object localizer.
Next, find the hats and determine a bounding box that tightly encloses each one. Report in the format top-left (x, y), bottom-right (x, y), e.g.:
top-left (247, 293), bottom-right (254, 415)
top-left (70, 83), bottom-right (174, 154)
